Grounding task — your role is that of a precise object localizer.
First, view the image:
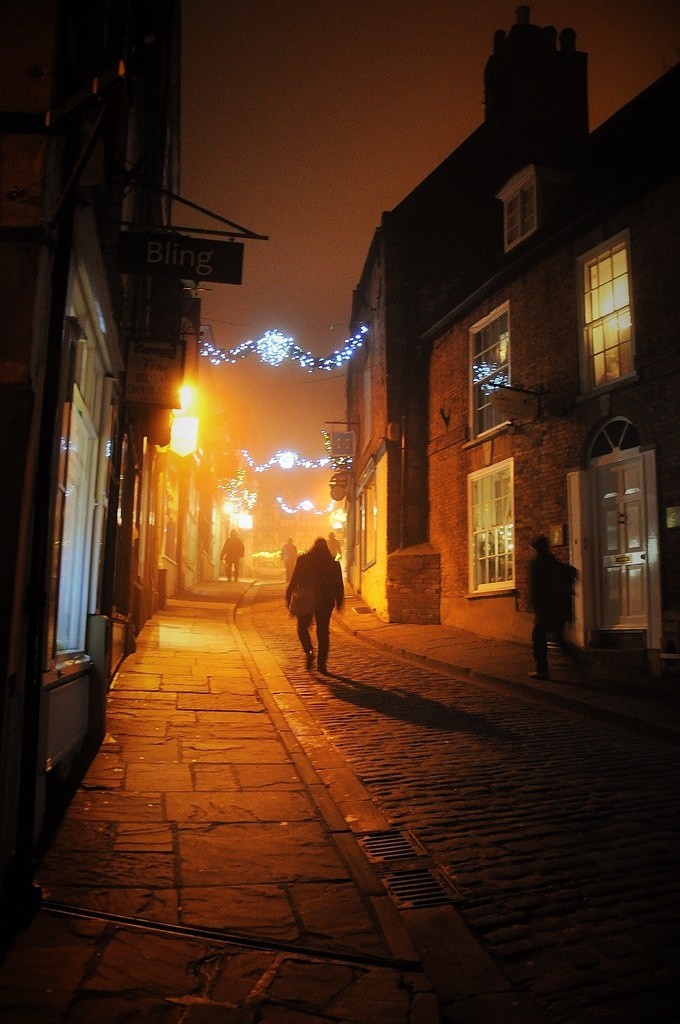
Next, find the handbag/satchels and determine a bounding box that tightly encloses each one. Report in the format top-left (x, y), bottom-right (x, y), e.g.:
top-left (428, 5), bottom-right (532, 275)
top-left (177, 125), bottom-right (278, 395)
top-left (289, 585), bottom-right (314, 617)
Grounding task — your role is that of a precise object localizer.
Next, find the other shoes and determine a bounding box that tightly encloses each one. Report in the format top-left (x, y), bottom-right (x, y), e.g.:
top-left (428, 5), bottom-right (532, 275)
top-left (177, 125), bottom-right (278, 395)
top-left (572, 654), bottom-right (587, 666)
top-left (528, 671), bottom-right (549, 681)
top-left (306, 653), bottom-right (314, 669)
top-left (228, 577), bottom-right (231, 581)
top-left (317, 664), bottom-right (327, 674)
top-left (234, 577), bottom-right (237, 582)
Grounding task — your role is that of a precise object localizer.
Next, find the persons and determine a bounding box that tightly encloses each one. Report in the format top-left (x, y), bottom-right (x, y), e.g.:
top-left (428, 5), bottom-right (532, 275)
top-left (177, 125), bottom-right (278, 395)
top-left (221, 530), bottom-right (245, 583)
top-left (326, 533), bottom-right (342, 560)
top-left (281, 537), bottom-right (298, 584)
top-left (286, 538), bottom-right (344, 672)
top-left (528, 534), bottom-right (587, 681)
